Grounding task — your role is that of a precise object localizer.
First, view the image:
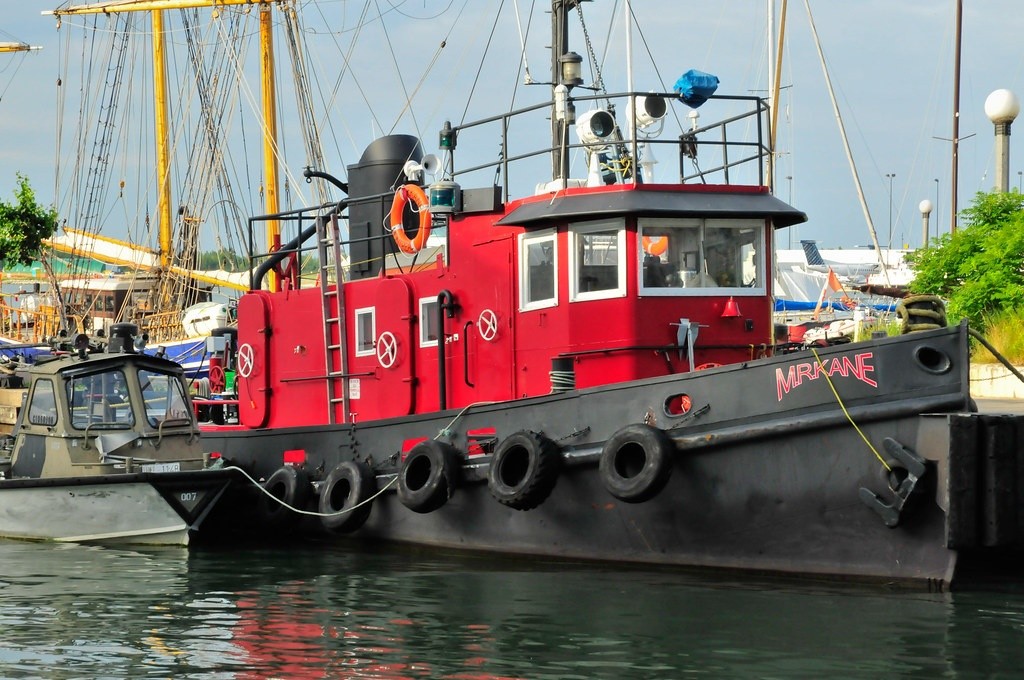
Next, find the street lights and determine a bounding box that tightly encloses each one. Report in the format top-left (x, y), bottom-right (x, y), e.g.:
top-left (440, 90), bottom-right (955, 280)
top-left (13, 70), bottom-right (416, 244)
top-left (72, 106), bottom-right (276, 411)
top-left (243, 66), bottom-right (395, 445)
top-left (985, 84), bottom-right (1021, 206)
top-left (884, 173), bottom-right (896, 248)
top-left (934, 178), bottom-right (940, 252)
top-left (1016, 171), bottom-right (1022, 205)
top-left (784, 175), bottom-right (793, 249)
top-left (917, 198), bottom-right (934, 249)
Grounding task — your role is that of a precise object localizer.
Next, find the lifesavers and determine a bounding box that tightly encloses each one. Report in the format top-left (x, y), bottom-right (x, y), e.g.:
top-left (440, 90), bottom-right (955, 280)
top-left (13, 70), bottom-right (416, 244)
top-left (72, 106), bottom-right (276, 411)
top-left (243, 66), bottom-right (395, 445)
top-left (390, 184), bottom-right (433, 255)
top-left (250, 423), bottom-right (677, 536)
top-left (642, 236), bottom-right (668, 255)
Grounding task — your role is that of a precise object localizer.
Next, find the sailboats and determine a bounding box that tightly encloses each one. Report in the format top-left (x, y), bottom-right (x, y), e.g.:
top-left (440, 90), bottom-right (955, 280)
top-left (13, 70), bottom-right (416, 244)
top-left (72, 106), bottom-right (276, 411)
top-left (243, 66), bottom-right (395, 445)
top-left (0, 0), bottom-right (333, 543)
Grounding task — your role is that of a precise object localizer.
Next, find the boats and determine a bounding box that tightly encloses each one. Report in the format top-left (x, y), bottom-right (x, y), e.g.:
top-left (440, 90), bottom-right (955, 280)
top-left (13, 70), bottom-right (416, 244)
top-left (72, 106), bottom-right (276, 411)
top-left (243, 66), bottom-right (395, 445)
top-left (91, 68), bottom-right (1024, 597)
